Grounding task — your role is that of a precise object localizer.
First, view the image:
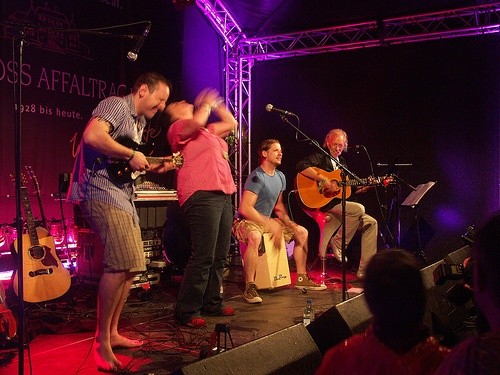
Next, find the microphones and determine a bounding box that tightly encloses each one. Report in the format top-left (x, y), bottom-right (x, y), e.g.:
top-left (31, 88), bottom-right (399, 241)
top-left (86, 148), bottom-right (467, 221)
top-left (344, 145), bottom-right (364, 151)
top-left (266, 104), bottom-right (296, 116)
top-left (127, 24), bottom-right (152, 62)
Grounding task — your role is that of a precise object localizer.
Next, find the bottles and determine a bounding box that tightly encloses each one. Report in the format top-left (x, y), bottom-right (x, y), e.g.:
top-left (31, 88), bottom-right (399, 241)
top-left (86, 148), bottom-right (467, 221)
top-left (303, 299), bottom-right (314, 327)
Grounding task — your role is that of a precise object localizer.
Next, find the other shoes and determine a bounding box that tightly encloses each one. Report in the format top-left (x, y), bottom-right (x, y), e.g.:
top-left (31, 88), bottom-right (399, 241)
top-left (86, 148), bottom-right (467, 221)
top-left (330, 237), bottom-right (348, 263)
top-left (217, 307), bottom-right (236, 316)
top-left (181, 317), bottom-right (205, 329)
top-left (356, 272), bottom-right (365, 282)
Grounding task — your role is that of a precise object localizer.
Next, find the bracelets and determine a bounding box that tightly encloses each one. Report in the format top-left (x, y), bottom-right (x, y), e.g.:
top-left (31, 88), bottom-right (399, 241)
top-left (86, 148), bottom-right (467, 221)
top-left (205, 104), bottom-right (211, 117)
top-left (318, 178), bottom-right (327, 190)
top-left (127, 151), bottom-right (135, 163)
top-left (288, 221), bottom-right (295, 227)
top-left (314, 173), bottom-right (321, 181)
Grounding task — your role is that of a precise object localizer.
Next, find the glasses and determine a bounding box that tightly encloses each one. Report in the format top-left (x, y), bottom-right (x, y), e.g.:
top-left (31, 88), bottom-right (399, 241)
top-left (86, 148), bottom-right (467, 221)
top-left (463, 256), bottom-right (483, 292)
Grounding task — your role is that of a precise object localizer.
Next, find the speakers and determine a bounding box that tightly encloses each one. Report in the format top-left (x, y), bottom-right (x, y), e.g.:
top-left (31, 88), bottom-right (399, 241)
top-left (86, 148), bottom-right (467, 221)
top-left (423, 244), bottom-right (473, 290)
top-left (171, 294), bottom-right (373, 375)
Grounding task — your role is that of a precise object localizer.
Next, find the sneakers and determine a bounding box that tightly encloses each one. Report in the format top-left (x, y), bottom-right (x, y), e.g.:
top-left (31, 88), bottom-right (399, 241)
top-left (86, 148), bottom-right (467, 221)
top-left (242, 282), bottom-right (262, 304)
top-left (293, 273), bottom-right (327, 291)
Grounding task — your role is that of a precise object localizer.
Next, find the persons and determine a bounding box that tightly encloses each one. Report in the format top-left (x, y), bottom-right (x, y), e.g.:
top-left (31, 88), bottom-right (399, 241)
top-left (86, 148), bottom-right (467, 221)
top-left (434, 209), bottom-right (500, 375)
top-left (318, 249), bottom-right (452, 375)
top-left (296, 129), bottom-right (393, 278)
top-left (161, 88), bottom-right (238, 329)
top-left (231, 139), bottom-right (328, 303)
top-left (66, 75), bottom-right (177, 371)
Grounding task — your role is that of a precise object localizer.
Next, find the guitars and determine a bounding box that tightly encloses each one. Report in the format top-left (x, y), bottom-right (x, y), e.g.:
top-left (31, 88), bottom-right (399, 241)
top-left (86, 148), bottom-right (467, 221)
top-left (294, 166), bottom-right (400, 210)
top-left (103, 137), bottom-right (185, 185)
top-left (25, 164), bottom-right (52, 235)
top-left (0, 292), bottom-right (17, 339)
top-left (9, 169), bottom-right (71, 303)
